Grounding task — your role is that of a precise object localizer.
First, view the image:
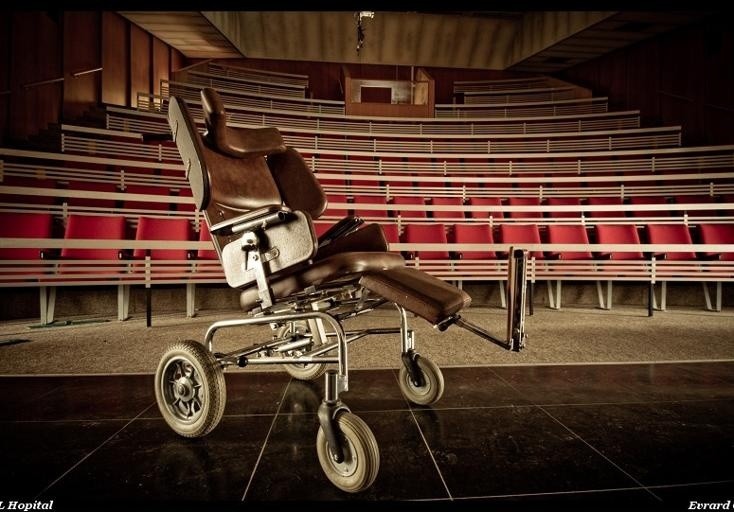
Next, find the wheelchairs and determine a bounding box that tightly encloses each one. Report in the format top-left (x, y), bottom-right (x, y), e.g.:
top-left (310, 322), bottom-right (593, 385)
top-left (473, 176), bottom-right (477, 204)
top-left (152, 87), bottom-right (530, 495)
